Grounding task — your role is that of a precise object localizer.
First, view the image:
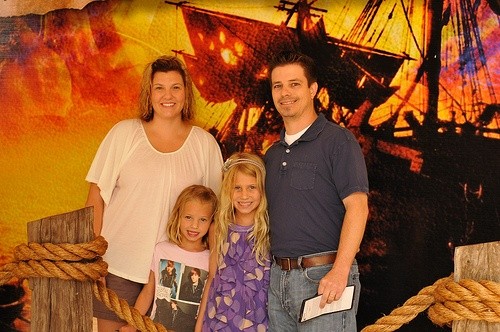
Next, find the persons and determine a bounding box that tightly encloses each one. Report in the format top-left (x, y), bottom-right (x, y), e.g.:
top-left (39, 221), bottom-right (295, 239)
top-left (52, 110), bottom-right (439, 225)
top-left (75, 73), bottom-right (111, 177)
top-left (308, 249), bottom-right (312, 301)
top-left (179, 267), bottom-right (205, 303)
top-left (193, 153), bottom-right (275, 332)
top-left (119, 184), bottom-right (220, 332)
top-left (161, 260), bottom-right (177, 289)
top-left (261, 50), bottom-right (371, 332)
top-left (82, 54), bottom-right (225, 332)
top-left (168, 300), bottom-right (182, 326)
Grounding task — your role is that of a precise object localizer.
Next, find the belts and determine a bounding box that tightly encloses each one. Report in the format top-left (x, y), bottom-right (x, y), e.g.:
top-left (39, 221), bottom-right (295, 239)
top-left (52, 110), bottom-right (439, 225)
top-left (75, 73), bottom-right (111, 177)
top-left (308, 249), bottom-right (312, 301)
top-left (271, 252), bottom-right (337, 271)
